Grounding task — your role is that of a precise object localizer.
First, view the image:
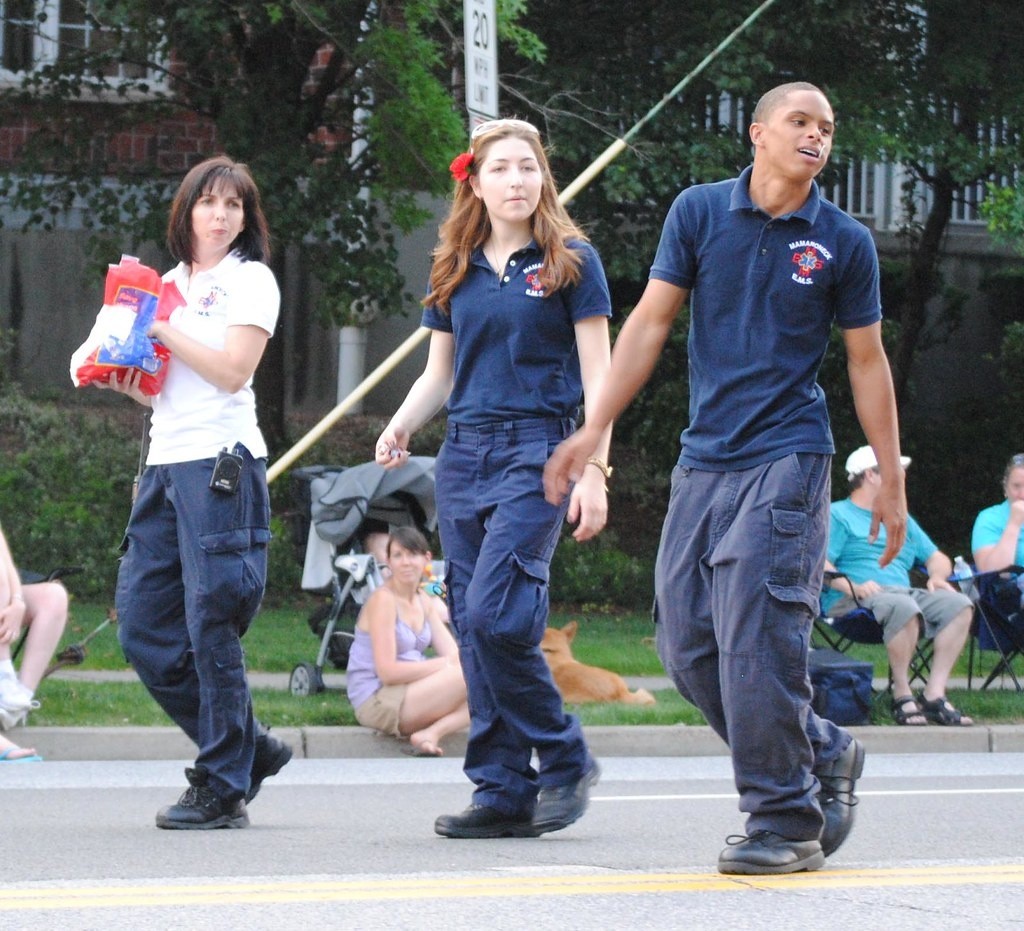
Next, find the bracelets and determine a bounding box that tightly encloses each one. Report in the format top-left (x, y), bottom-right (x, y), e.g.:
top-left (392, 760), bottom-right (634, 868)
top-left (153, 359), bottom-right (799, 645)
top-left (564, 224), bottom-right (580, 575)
top-left (585, 457), bottom-right (613, 479)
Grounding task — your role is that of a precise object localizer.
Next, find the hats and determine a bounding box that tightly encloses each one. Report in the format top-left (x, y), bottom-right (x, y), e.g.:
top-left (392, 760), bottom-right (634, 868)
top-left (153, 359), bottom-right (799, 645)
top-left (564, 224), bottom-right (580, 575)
top-left (844, 445), bottom-right (912, 482)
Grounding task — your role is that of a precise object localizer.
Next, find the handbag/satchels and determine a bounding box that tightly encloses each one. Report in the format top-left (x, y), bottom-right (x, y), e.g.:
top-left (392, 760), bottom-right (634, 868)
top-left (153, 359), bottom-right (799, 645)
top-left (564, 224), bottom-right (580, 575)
top-left (807, 648), bottom-right (874, 726)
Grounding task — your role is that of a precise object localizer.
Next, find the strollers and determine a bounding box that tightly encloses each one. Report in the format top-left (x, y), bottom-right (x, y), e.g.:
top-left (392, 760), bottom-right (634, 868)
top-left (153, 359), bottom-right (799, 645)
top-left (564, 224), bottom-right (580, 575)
top-left (287, 462), bottom-right (434, 699)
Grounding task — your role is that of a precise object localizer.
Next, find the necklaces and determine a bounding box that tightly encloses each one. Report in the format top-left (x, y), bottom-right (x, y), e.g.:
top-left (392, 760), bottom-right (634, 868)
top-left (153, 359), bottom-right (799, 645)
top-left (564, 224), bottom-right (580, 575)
top-left (492, 238), bottom-right (506, 276)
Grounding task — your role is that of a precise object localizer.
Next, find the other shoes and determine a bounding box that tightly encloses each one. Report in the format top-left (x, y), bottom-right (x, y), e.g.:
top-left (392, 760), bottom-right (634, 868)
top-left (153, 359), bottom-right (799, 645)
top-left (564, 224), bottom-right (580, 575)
top-left (1, 668), bottom-right (32, 730)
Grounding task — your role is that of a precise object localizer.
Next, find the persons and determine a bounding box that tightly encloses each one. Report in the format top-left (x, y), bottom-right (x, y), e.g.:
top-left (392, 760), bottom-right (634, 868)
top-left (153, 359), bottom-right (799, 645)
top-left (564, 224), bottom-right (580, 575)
top-left (98, 158), bottom-right (293, 831)
top-left (348, 527), bottom-right (470, 758)
top-left (542, 81), bottom-right (906, 875)
top-left (0, 530), bottom-right (68, 763)
top-left (375, 118), bottom-right (617, 839)
top-left (819, 444), bottom-right (974, 726)
top-left (971, 454), bottom-right (1024, 624)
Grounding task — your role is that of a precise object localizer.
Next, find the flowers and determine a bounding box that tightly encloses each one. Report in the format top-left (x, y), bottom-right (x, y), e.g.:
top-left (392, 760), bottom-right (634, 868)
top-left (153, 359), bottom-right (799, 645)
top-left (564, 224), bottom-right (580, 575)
top-left (450, 153), bottom-right (476, 182)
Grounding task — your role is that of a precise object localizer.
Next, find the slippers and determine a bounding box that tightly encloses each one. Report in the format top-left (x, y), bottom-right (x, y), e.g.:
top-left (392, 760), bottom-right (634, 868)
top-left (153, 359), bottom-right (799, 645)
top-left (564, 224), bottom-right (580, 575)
top-left (0, 744), bottom-right (43, 761)
top-left (400, 741), bottom-right (441, 758)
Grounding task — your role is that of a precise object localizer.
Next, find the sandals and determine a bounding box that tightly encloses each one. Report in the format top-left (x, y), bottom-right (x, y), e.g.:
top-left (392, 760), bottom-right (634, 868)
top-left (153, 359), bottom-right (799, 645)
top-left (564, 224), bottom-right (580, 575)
top-left (890, 692), bottom-right (929, 726)
top-left (918, 687), bottom-right (973, 726)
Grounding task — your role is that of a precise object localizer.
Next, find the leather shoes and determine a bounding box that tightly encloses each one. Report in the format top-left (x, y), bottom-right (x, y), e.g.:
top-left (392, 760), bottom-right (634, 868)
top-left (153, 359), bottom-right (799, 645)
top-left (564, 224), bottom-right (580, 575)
top-left (718, 831), bottom-right (826, 876)
top-left (815, 738), bottom-right (866, 859)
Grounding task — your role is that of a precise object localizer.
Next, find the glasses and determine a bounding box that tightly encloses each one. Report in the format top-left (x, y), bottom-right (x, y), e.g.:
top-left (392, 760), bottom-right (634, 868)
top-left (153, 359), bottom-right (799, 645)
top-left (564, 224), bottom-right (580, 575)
top-left (1011, 455), bottom-right (1024, 465)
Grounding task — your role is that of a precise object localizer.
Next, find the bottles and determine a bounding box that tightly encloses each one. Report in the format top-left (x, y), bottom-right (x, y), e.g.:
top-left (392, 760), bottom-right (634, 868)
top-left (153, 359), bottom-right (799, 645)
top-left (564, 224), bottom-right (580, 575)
top-left (953, 555), bottom-right (981, 602)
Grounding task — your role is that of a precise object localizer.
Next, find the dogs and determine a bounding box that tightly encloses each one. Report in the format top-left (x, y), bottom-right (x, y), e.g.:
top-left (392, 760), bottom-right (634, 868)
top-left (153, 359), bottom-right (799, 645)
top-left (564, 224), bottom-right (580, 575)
top-left (537, 620), bottom-right (657, 702)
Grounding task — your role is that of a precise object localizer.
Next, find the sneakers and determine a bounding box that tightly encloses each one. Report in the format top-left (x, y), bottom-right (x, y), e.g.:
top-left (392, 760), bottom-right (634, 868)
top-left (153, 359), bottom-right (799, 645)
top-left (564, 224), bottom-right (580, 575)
top-left (530, 751), bottom-right (599, 834)
top-left (244, 723), bottom-right (292, 807)
top-left (435, 801), bottom-right (540, 839)
top-left (155, 768), bottom-right (250, 829)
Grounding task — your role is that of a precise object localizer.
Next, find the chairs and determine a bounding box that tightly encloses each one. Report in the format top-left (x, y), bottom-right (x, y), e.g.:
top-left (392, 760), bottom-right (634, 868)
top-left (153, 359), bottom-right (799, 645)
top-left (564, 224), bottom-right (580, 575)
top-left (808, 572), bottom-right (939, 712)
top-left (946, 562), bottom-right (1024, 695)
